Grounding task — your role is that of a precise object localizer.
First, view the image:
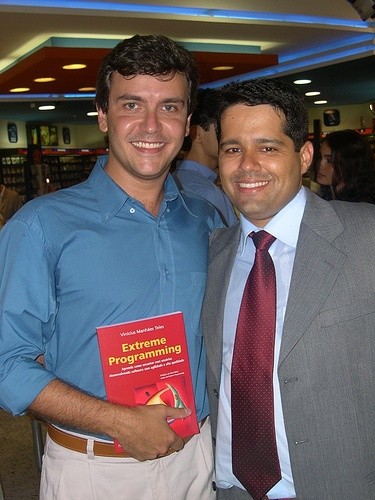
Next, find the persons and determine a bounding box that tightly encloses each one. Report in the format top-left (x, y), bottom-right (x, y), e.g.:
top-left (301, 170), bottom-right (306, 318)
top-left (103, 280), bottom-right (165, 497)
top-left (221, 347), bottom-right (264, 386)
top-left (0, 183), bottom-right (22, 229)
top-left (0, 33), bottom-right (245, 500)
top-left (169, 87), bottom-right (240, 229)
top-left (316, 129), bottom-right (375, 207)
top-left (199, 78), bottom-right (375, 500)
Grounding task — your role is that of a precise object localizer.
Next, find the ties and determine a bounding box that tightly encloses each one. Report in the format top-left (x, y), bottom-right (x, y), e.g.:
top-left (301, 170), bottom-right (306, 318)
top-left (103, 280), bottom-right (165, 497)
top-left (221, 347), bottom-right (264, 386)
top-left (231, 229), bottom-right (281, 498)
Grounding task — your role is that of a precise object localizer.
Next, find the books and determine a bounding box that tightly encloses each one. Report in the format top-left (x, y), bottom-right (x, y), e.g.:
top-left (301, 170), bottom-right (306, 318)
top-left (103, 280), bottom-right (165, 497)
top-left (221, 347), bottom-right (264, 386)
top-left (96, 311), bottom-right (200, 453)
top-left (0, 156), bottom-right (97, 201)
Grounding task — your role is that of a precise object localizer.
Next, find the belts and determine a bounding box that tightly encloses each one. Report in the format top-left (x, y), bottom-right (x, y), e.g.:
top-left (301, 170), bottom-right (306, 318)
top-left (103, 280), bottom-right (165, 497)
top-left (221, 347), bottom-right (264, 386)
top-left (45, 416), bottom-right (207, 458)
